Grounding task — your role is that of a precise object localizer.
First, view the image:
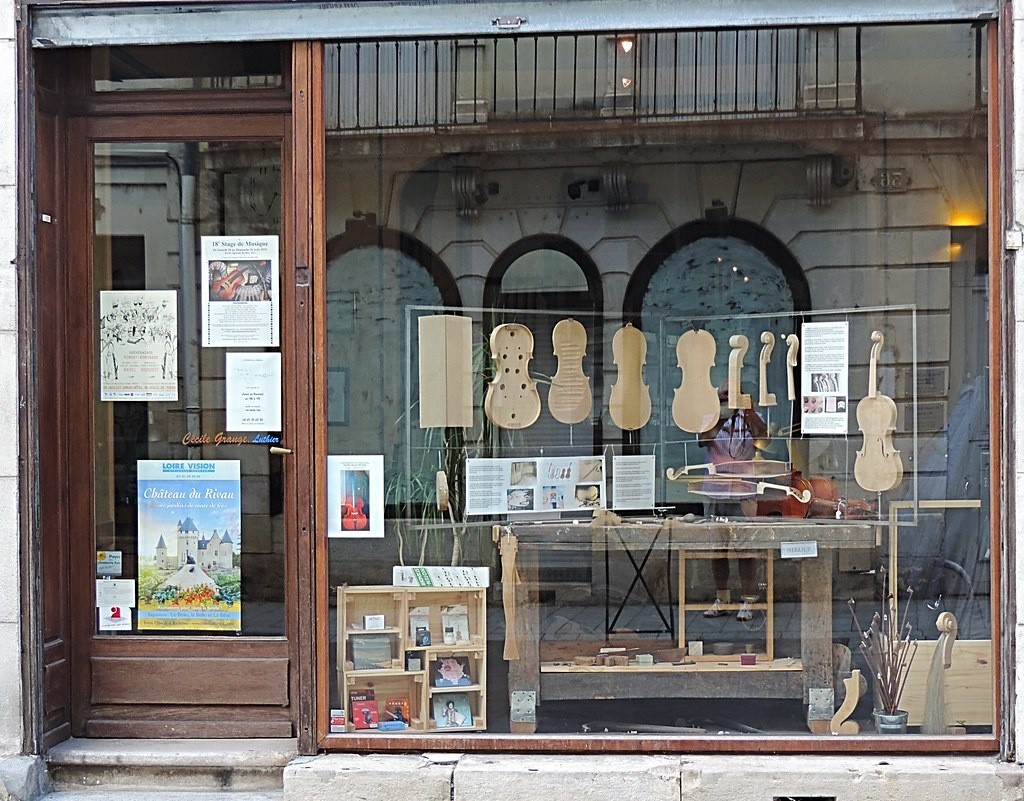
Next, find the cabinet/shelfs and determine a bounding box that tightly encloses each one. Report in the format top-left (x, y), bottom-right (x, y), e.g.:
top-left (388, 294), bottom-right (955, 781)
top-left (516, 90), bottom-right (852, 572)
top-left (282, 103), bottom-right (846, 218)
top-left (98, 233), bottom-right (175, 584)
top-left (335, 583), bottom-right (488, 735)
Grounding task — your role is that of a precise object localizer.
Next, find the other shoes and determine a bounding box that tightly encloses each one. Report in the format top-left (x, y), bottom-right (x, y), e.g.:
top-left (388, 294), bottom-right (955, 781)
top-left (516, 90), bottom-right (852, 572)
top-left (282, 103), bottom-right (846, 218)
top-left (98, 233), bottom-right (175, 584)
top-left (703, 597), bottom-right (732, 617)
top-left (737, 603), bottom-right (755, 621)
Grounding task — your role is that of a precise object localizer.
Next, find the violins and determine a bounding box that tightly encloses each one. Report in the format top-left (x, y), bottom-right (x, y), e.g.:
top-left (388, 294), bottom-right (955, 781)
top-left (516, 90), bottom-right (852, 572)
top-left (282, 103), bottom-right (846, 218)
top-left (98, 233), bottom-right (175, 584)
top-left (210, 261), bottom-right (264, 300)
top-left (853, 329), bottom-right (904, 492)
top-left (341, 471), bottom-right (369, 531)
top-left (737, 472), bottom-right (884, 524)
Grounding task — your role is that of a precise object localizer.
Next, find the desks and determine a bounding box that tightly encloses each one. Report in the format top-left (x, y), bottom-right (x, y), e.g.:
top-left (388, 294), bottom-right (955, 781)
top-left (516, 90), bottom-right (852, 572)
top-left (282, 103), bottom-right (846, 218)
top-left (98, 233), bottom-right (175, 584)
top-left (491, 515), bottom-right (883, 737)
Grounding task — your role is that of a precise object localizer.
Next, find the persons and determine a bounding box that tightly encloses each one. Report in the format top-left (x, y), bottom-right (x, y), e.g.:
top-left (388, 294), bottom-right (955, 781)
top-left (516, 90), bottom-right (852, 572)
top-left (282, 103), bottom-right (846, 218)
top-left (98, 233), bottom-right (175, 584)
top-left (698, 380), bottom-right (772, 621)
